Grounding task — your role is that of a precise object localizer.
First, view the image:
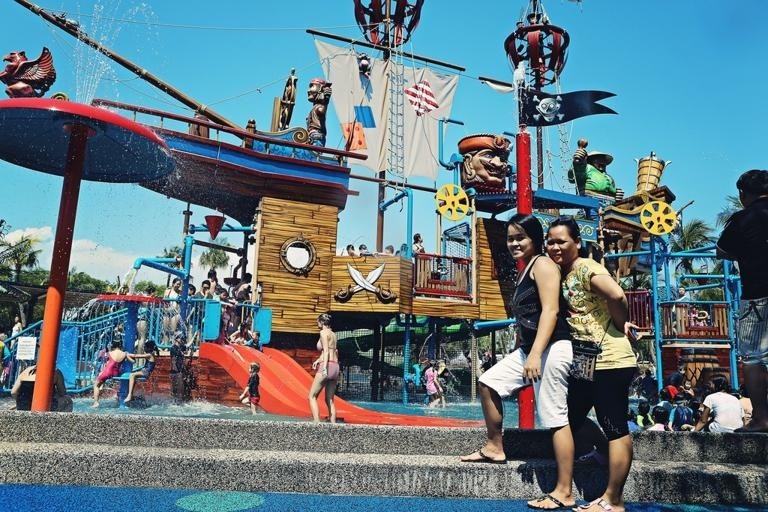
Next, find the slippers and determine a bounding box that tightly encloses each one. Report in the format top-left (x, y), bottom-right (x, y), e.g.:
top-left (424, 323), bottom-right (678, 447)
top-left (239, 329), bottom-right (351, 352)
top-left (572, 497), bottom-right (614, 512)
top-left (573, 450), bottom-right (609, 466)
top-left (527, 494), bottom-right (577, 510)
top-left (461, 450), bottom-right (507, 464)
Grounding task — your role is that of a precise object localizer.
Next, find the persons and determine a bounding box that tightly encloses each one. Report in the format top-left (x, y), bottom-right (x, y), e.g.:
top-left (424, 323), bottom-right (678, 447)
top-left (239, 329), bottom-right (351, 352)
top-left (461, 214), bottom-right (579, 508)
top-left (308, 313), bottom-right (340, 423)
top-left (240, 361), bottom-right (260, 415)
top-left (168, 330), bottom-right (197, 404)
top-left (424, 359), bottom-right (444, 410)
top-left (11, 365), bottom-right (75, 413)
top-left (715, 168), bottom-right (767, 431)
top-left (10, 314), bottom-right (23, 348)
top-left (121, 339), bottom-right (158, 403)
top-left (672, 286), bottom-right (686, 338)
top-left (358, 244), bottom-right (367, 254)
top-left (131, 269), bottom-right (263, 370)
top-left (90, 341), bottom-right (136, 408)
top-left (347, 245), bottom-right (357, 256)
top-left (413, 232), bottom-right (426, 297)
top-left (386, 244), bottom-right (393, 254)
top-left (432, 263), bottom-right (449, 298)
top-left (621, 368), bottom-right (755, 434)
top-left (0, 332), bottom-right (10, 391)
top-left (3, 358), bottom-right (16, 389)
top-left (545, 214), bottom-right (639, 512)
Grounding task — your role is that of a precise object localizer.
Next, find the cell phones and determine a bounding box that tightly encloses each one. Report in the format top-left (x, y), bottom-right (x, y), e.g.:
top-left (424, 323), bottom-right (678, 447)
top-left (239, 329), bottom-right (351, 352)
top-left (628, 327), bottom-right (637, 340)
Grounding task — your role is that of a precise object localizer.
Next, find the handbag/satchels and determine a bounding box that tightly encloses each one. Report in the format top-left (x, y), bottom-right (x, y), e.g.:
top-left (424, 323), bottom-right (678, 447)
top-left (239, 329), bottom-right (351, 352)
top-left (569, 339), bottom-right (602, 382)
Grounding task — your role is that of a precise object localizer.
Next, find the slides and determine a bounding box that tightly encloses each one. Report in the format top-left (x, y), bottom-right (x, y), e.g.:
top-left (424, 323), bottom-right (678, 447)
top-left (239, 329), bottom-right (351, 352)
top-left (199, 338), bottom-right (392, 420)
top-left (336, 317), bottom-right (490, 386)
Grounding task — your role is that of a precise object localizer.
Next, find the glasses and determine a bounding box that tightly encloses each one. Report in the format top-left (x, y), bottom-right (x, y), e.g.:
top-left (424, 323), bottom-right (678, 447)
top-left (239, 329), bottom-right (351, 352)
top-left (549, 215), bottom-right (579, 233)
